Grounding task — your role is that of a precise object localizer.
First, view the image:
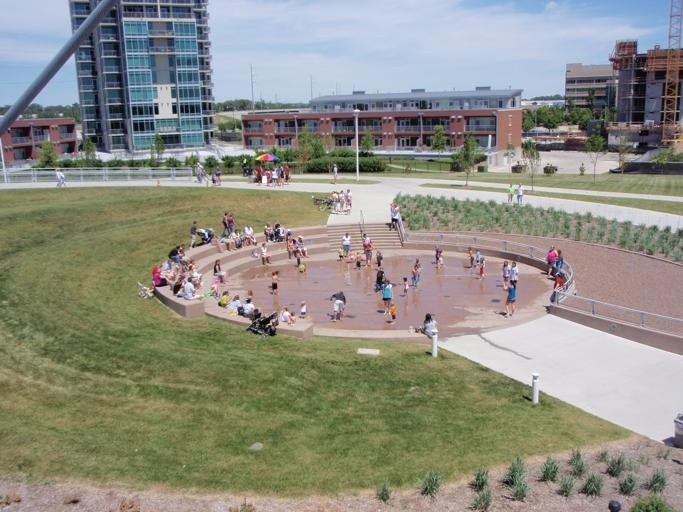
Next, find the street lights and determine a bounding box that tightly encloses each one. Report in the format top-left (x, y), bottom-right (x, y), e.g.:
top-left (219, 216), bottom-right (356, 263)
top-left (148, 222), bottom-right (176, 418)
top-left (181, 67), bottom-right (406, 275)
top-left (351, 108), bottom-right (363, 182)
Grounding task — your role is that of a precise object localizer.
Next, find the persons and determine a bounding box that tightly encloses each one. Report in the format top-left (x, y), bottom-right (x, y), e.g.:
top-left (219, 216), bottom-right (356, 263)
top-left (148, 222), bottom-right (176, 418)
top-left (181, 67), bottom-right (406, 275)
top-left (194, 162), bottom-right (290, 186)
top-left (330, 292), bottom-right (346, 322)
top-left (390, 202), bottom-right (400, 230)
top-left (332, 189), bottom-right (352, 215)
top-left (190, 221), bottom-right (215, 248)
top-left (332, 164), bottom-right (338, 183)
top-left (210, 259), bottom-right (228, 297)
top-left (411, 258), bottom-right (423, 288)
top-left (282, 301), bottom-right (307, 325)
top-left (501, 260), bottom-right (520, 318)
top-left (467, 247), bottom-right (485, 280)
top-left (402, 277), bottom-right (410, 298)
top-left (218, 289), bottom-right (263, 321)
top-left (339, 232), bottom-right (398, 325)
top-left (545, 245), bottom-right (567, 302)
top-left (435, 245), bottom-right (443, 270)
top-left (271, 270), bottom-right (280, 296)
top-left (507, 184), bottom-right (523, 204)
top-left (59, 169), bottom-right (66, 188)
top-left (415, 313), bottom-right (439, 338)
top-left (55, 168), bottom-right (61, 188)
top-left (152, 244), bottom-right (203, 300)
top-left (220, 210), bottom-right (259, 257)
top-left (261, 222), bottom-right (310, 273)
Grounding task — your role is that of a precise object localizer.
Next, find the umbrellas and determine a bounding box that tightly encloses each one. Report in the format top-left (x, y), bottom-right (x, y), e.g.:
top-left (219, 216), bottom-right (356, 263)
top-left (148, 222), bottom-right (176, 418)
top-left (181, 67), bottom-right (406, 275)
top-left (255, 152), bottom-right (280, 161)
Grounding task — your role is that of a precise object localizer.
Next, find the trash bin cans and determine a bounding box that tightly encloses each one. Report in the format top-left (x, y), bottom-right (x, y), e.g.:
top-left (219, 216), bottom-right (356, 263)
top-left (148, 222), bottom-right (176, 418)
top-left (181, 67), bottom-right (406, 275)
top-left (674, 413), bottom-right (683, 448)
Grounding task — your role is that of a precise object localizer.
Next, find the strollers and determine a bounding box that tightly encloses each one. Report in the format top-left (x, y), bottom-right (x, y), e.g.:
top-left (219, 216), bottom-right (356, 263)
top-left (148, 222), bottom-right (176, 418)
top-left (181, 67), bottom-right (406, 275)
top-left (197, 229), bottom-right (216, 243)
top-left (134, 280), bottom-right (155, 300)
top-left (245, 311), bottom-right (279, 337)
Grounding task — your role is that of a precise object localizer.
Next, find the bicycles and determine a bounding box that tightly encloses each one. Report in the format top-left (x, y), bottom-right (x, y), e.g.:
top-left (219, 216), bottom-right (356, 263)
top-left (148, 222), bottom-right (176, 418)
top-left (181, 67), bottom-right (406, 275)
top-left (317, 197), bottom-right (334, 212)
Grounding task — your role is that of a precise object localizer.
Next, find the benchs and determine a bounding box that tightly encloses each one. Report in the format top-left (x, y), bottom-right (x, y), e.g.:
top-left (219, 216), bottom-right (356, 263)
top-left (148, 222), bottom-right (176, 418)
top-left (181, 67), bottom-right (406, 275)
top-left (151, 225), bottom-right (331, 338)
